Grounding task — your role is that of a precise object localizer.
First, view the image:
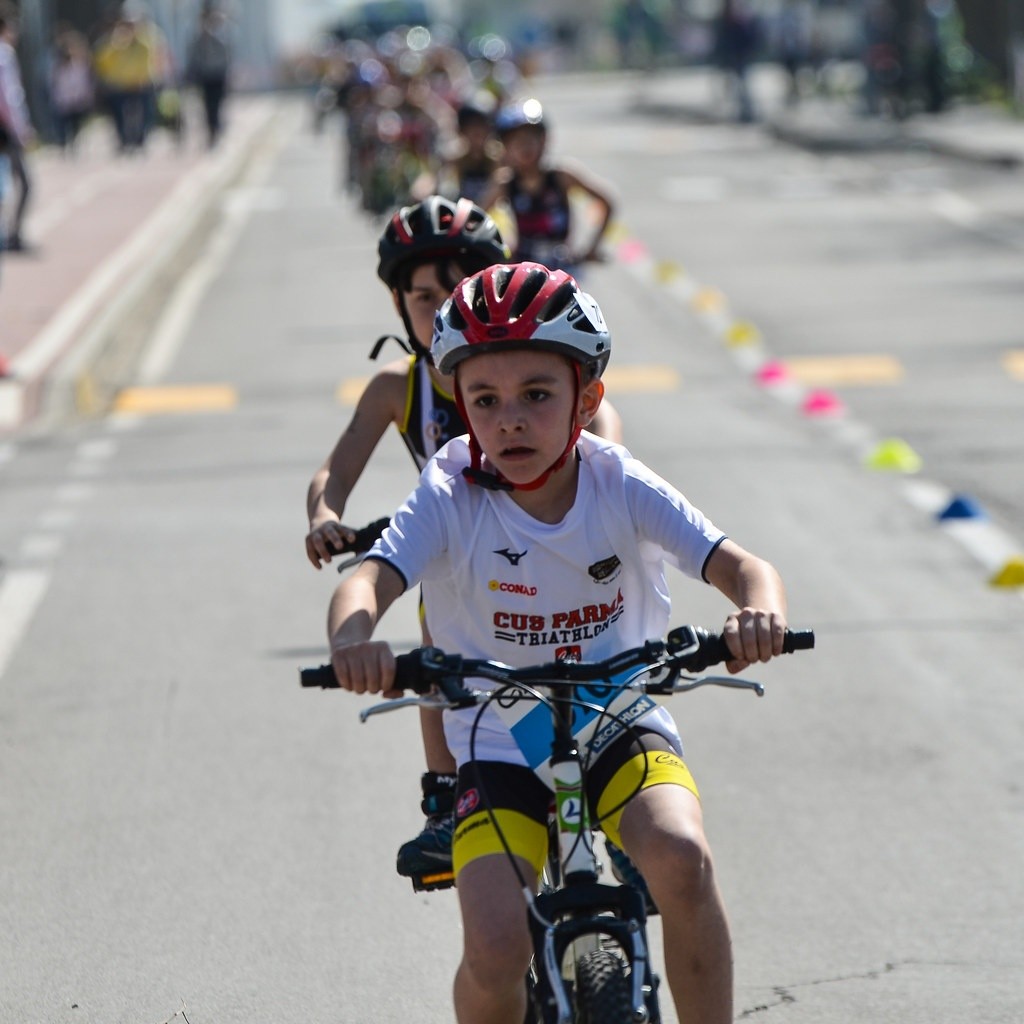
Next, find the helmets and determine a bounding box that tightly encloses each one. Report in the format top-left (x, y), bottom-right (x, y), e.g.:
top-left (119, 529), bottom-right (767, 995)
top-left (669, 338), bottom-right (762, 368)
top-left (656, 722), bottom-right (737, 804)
top-left (378, 195), bottom-right (510, 287)
top-left (431, 263), bottom-right (609, 392)
top-left (493, 102), bottom-right (548, 144)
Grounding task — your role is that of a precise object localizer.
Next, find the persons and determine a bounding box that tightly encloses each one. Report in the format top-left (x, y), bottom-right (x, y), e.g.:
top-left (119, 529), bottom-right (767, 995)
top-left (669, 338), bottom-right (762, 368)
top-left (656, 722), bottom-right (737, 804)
top-left (46, 3), bottom-right (243, 165)
top-left (325, 262), bottom-right (789, 1024)
top-left (307, 23), bottom-right (618, 300)
top-left (303, 198), bottom-right (624, 879)
top-left (622, 0), bottom-right (1016, 132)
top-left (0, 12), bottom-right (43, 257)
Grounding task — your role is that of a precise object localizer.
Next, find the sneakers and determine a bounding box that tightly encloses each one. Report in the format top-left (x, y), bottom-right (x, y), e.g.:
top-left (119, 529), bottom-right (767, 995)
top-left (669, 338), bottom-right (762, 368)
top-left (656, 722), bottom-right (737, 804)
top-left (609, 838), bottom-right (661, 916)
top-left (396, 770), bottom-right (461, 871)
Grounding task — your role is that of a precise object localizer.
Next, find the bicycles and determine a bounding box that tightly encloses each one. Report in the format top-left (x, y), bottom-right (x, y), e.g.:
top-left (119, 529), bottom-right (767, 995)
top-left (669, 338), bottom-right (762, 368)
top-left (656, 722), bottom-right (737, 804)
top-left (301, 627), bottom-right (817, 1024)
